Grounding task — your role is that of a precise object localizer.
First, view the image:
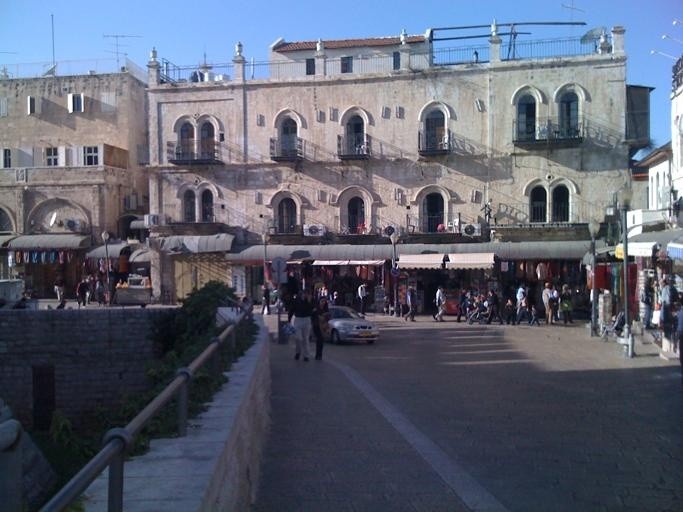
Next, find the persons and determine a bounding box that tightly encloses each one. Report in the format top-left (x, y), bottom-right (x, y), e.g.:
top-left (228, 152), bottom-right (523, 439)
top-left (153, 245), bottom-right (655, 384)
top-left (400, 283), bottom-right (419, 322)
top-left (260, 270), bottom-right (370, 362)
top-left (52, 268), bottom-right (107, 307)
top-left (432, 282), bottom-right (577, 327)
top-left (640, 274), bottom-right (682, 371)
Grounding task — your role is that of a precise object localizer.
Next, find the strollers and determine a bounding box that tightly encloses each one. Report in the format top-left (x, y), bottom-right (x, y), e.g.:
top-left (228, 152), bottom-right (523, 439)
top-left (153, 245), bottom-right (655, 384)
top-left (599, 311), bottom-right (625, 343)
top-left (465, 302), bottom-right (488, 324)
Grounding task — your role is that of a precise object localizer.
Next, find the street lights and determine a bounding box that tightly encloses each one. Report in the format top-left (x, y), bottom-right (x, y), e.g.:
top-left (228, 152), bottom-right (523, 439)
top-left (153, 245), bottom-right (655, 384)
top-left (616, 182), bottom-right (633, 359)
top-left (101, 230), bottom-right (111, 304)
top-left (588, 218), bottom-right (600, 338)
top-left (389, 232), bottom-right (398, 317)
top-left (261, 232), bottom-right (270, 305)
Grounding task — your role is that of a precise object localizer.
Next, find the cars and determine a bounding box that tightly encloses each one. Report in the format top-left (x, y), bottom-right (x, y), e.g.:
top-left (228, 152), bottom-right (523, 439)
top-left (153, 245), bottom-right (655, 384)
top-left (308, 305), bottom-right (379, 344)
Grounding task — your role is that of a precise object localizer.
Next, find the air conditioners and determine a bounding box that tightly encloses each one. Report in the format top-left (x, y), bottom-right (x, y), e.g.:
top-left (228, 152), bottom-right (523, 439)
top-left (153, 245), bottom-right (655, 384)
top-left (460, 222), bottom-right (482, 238)
top-left (380, 224), bottom-right (399, 237)
top-left (302, 223), bottom-right (325, 237)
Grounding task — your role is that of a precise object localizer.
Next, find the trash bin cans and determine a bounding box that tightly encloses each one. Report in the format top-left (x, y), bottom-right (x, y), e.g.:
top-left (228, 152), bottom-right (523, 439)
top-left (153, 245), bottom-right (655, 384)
top-left (279, 320), bottom-right (290, 343)
